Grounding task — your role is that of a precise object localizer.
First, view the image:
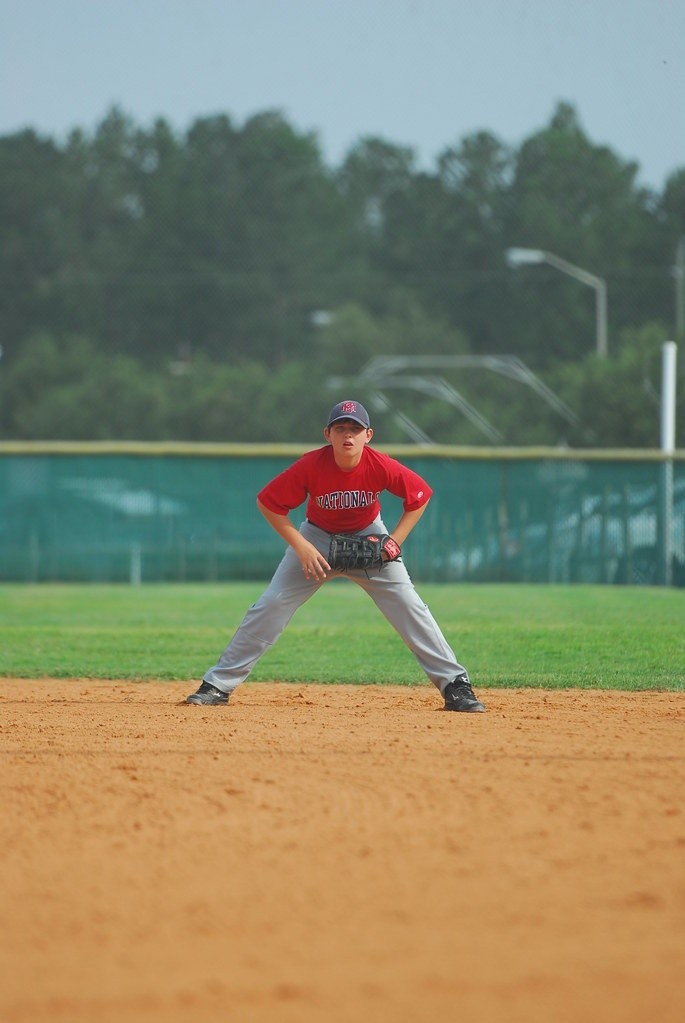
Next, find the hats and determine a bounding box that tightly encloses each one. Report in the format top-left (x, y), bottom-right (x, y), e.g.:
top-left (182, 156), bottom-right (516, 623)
top-left (327, 400), bottom-right (369, 430)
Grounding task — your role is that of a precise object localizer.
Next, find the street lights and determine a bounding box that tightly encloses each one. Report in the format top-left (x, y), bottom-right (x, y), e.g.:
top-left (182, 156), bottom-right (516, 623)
top-left (505, 245), bottom-right (608, 360)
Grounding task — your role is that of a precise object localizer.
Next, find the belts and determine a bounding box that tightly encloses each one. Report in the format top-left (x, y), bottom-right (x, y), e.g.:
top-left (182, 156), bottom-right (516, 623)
top-left (306, 519), bottom-right (333, 535)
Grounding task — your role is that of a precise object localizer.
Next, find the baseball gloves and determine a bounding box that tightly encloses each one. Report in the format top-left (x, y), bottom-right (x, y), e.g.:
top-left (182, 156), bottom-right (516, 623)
top-left (322, 532), bottom-right (403, 581)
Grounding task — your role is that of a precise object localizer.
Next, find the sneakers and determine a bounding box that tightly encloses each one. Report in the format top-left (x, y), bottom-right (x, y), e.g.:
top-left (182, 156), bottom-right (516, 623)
top-left (444, 679), bottom-right (484, 713)
top-left (186, 680), bottom-right (228, 706)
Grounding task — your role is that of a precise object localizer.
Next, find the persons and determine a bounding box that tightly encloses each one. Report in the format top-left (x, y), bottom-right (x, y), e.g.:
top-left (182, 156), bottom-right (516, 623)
top-left (186, 401), bottom-right (486, 712)
